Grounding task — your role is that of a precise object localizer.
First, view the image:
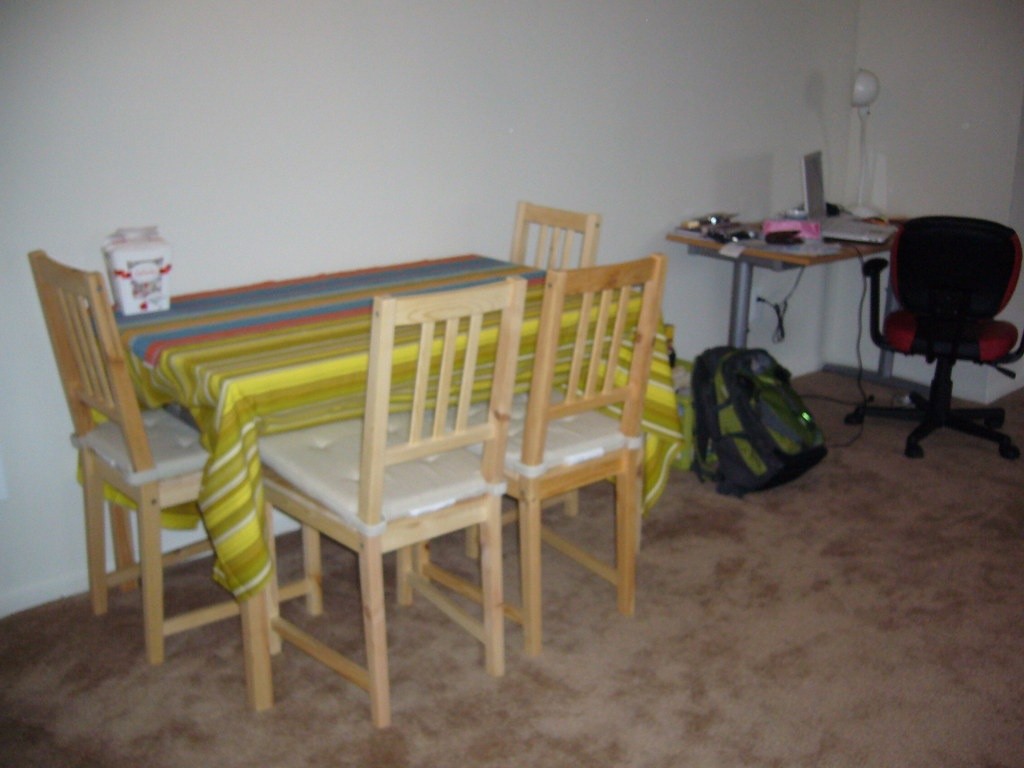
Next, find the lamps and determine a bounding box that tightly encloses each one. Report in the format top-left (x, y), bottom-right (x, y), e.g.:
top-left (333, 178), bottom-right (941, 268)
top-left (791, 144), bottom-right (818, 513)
top-left (847, 67), bottom-right (880, 218)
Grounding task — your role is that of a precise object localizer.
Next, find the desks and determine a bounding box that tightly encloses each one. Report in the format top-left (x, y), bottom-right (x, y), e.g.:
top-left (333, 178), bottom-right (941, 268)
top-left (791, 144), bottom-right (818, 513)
top-left (664, 218), bottom-right (930, 398)
top-left (75, 255), bottom-right (685, 712)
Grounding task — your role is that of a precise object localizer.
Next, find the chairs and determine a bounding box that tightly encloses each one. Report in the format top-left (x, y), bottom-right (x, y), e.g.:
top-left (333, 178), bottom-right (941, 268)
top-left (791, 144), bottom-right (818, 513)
top-left (463, 199), bottom-right (603, 560)
top-left (26, 249), bottom-right (323, 669)
top-left (259, 273), bottom-right (529, 727)
top-left (841, 216), bottom-right (1024, 462)
top-left (410, 251), bottom-right (669, 660)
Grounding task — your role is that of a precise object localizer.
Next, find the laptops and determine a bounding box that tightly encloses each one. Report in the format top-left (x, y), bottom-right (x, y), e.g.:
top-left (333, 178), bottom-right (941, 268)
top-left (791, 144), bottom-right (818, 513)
top-left (804, 150), bottom-right (898, 246)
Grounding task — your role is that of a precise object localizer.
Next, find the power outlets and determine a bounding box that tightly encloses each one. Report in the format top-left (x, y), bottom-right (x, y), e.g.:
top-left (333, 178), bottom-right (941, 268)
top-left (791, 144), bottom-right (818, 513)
top-left (751, 291), bottom-right (765, 318)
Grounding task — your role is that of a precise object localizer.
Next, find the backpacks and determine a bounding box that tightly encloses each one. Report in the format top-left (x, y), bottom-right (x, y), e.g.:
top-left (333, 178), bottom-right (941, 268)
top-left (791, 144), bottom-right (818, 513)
top-left (692, 346), bottom-right (828, 497)
